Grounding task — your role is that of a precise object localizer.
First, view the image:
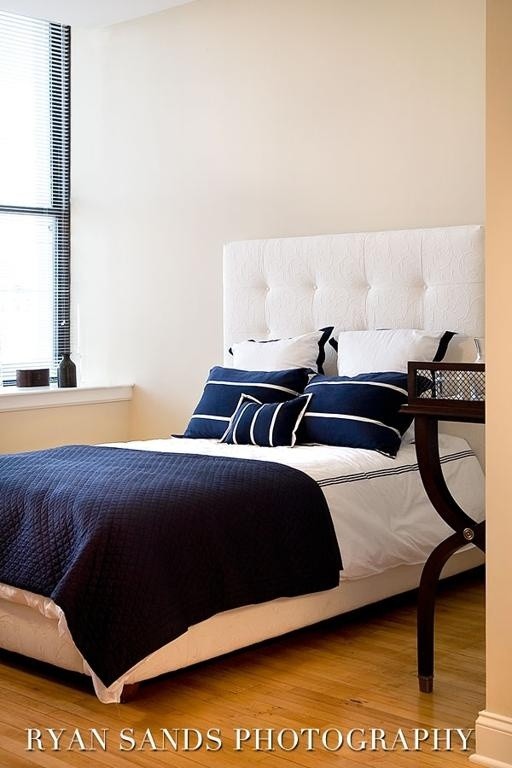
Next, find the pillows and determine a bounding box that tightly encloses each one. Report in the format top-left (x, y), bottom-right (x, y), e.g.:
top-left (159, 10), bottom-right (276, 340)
top-left (297, 372), bottom-right (435, 459)
top-left (170, 365), bottom-right (320, 439)
top-left (329, 329), bottom-right (457, 445)
top-left (217, 393), bottom-right (313, 448)
top-left (226, 326), bottom-right (334, 385)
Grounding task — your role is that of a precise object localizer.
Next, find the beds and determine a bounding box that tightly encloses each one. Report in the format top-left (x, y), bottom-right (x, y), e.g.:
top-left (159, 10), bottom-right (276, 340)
top-left (0, 223), bottom-right (487, 704)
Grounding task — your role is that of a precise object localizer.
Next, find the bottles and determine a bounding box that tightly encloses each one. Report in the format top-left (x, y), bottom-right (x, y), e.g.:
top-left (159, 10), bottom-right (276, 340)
top-left (57, 352), bottom-right (77, 387)
top-left (468, 338), bottom-right (485, 399)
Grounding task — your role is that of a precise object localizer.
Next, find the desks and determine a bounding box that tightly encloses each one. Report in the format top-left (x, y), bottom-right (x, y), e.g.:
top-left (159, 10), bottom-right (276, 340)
top-left (400, 360), bottom-right (485, 693)
